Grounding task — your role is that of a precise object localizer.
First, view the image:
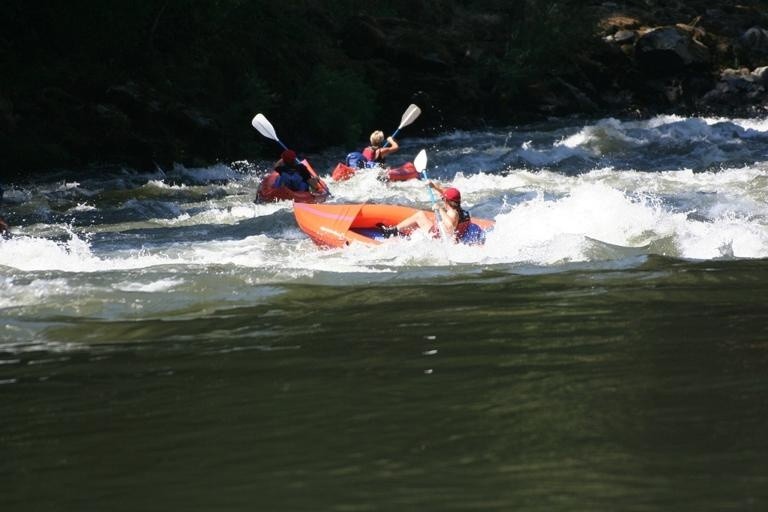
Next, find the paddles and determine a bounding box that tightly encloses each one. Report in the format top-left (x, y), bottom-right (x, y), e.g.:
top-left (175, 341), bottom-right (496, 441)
top-left (251, 114), bottom-right (300, 163)
top-left (413, 149), bottom-right (446, 238)
top-left (381, 104), bottom-right (422, 147)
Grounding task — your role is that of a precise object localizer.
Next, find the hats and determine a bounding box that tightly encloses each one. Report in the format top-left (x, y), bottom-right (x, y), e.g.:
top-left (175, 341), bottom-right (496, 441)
top-left (281, 149), bottom-right (295, 162)
top-left (441, 188), bottom-right (461, 204)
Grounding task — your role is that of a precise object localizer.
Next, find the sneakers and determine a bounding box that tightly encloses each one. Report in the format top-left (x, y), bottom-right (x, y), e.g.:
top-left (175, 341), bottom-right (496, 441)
top-left (375, 222), bottom-right (398, 239)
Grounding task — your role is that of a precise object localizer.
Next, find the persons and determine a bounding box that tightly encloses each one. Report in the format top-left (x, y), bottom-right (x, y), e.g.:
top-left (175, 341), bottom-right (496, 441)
top-left (273, 150), bottom-right (318, 194)
top-left (378, 181), bottom-right (471, 242)
top-left (360, 128), bottom-right (399, 168)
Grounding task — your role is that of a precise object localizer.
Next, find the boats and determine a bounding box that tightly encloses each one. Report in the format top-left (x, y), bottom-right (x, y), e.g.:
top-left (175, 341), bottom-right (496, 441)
top-left (330, 162), bottom-right (418, 186)
top-left (290, 198), bottom-right (496, 253)
top-left (253, 169), bottom-right (328, 205)
